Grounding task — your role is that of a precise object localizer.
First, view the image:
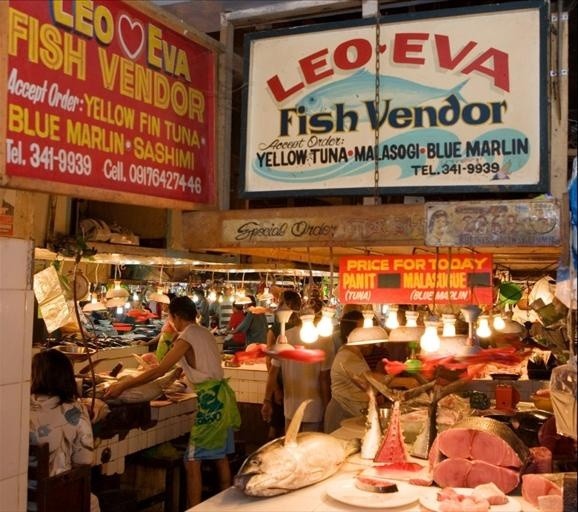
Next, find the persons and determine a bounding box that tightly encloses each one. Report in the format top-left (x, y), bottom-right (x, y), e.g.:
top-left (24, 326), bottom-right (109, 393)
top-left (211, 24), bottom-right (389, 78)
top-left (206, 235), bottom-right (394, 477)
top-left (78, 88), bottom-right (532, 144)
top-left (101, 283), bottom-right (418, 509)
top-left (28, 349), bottom-right (96, 512)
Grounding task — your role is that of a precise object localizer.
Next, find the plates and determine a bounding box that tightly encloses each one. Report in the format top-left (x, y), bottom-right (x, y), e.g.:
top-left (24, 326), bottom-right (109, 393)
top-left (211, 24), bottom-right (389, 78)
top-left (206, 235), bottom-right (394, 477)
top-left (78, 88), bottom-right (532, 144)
top-left (418, 487), bottom-right (523, 512)
top-left (489, 371), bottom-right (520, 381)
top-left (326, 475), bottom-right (418, 512)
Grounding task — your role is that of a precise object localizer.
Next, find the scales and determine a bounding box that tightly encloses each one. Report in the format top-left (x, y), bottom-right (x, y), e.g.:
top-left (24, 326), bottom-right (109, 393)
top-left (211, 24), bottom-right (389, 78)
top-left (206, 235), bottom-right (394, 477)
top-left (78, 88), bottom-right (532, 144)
top-left (491, 373), bottom-right (522, 410)
top-left (53, 345), bottom-right (96, 397)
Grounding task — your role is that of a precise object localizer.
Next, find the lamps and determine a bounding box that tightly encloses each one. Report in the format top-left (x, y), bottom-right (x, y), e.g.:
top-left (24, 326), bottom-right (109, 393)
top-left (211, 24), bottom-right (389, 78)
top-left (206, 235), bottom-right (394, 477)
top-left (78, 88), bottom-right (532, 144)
top-left (389, 302), bottom-right (426, 342)
top-left (83, 263), bottom-right (129, 312)
top-left (234, 272), bottom-right (252, 304)
top-left (149, 266), bottom-right (171, 305)
top-left (258, 272), bottom-right (273, 301)
top-left (344, 305), bottom-right (387, 347)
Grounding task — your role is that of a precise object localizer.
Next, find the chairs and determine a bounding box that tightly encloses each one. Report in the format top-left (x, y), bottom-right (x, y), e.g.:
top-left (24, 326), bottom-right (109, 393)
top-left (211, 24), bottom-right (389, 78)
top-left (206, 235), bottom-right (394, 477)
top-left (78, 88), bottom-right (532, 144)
top-left (28, 441), bottom-right (91, 511)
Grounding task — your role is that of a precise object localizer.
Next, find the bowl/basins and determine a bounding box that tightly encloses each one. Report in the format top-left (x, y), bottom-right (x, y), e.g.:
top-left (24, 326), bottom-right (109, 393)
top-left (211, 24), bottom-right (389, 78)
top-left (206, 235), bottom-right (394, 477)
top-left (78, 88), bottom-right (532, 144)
top-left (497, 282), bottom-right (521, 306)
top-left (98, 318), bottom-right (111, 325)
top-left (379, 408), bottom-right (391, 429)
top-left (53, 344), bottom-right (96, 362)
top-left (530, 389), bottom-right (555, 411)
